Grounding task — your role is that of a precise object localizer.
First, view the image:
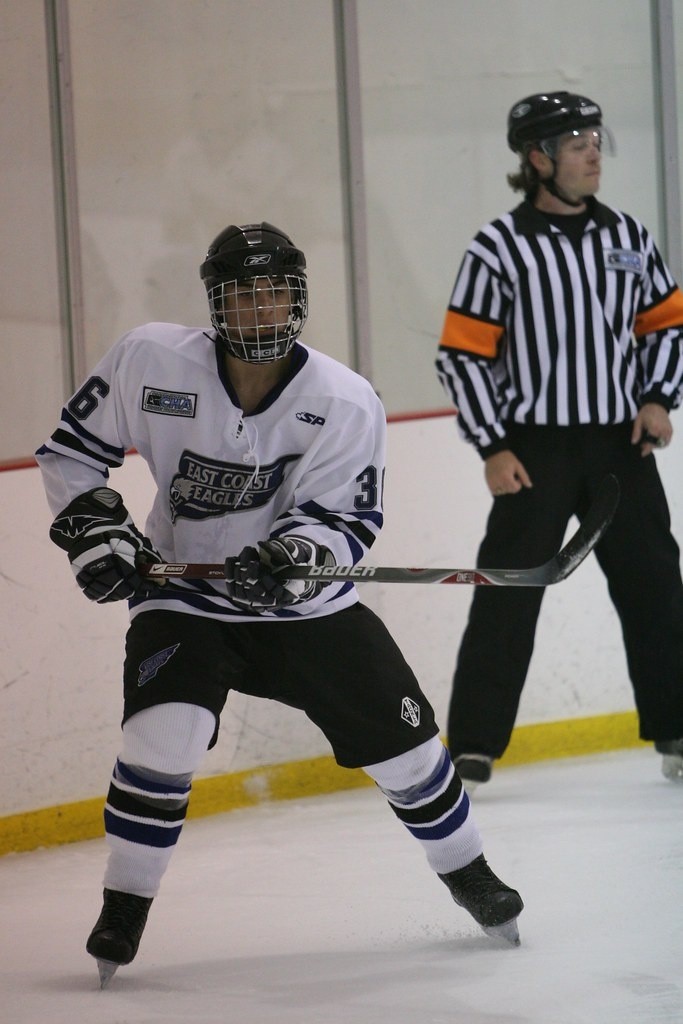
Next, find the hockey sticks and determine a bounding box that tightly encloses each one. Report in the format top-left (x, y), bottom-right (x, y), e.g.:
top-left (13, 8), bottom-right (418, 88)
top-left (141, 476), bottom-right (626, 590)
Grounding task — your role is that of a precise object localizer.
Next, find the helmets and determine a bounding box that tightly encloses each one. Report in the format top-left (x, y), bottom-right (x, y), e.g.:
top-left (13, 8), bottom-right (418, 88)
top-left (200, 221), bottom-right (308, 366)
top-left (508, 91), bottom-right (603, 154)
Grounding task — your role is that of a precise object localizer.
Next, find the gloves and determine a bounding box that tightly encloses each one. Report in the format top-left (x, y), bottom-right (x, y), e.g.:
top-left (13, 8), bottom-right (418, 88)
top-left (225, 535), bottom-right (337, 615)
top-left (49, 487), bottom-right (169, 604)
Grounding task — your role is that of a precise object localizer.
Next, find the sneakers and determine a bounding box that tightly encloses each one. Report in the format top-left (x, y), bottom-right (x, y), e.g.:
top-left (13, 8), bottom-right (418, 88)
top-left (438, 852), bottom-right (524, 946)
top-left (452, 754), bottom-right (492, 796)
top-left (654, 738), bottom-right (683, 780)
top-left (86, 887), bottom-right (154, 992)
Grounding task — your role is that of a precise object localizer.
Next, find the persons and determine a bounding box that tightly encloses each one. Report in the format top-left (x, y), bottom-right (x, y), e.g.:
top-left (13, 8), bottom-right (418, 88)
top-left (443, 90), bottom-right (683, 789)
top-left (34, 222), bottom-right (525, 987)
